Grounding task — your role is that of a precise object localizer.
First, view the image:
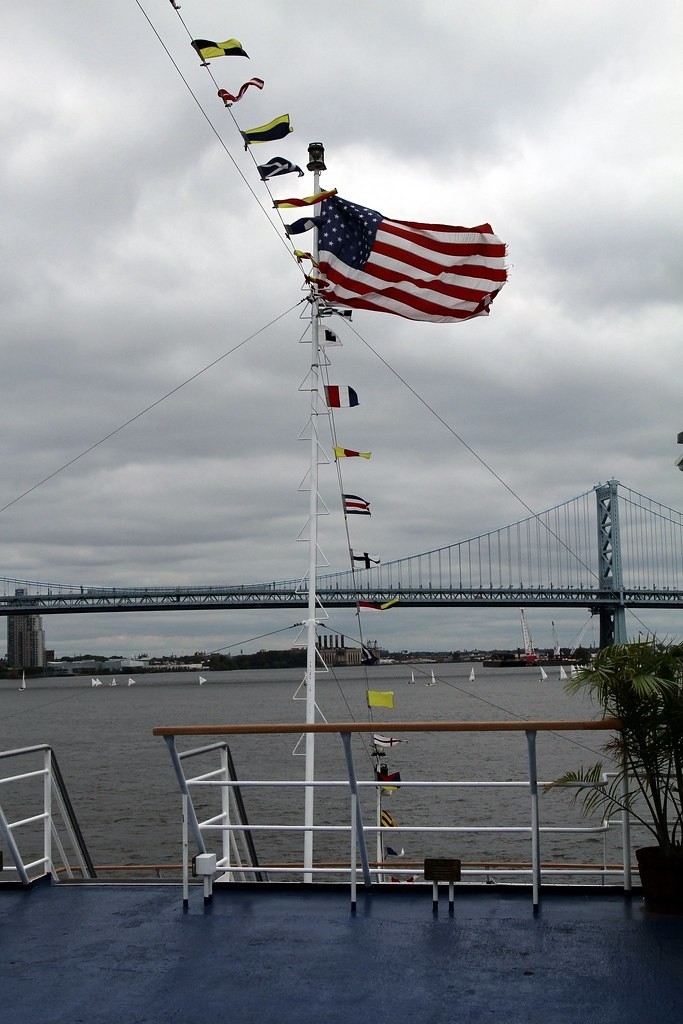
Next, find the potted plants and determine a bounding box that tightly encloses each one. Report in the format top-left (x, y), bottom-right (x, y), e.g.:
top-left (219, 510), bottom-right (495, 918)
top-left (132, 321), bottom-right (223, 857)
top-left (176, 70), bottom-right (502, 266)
top-left (539, 636), bottom-right (683, 914)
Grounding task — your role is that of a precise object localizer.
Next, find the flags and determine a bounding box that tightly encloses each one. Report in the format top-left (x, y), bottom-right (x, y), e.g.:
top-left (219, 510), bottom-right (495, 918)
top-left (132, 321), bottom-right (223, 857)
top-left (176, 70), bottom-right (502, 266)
top-left (315, 187), bottom-right (508, 324)
top-left (362, 646), bottom-right (421, 882)
top-left (324, 385), bottom-right (358, 407)
top-left (272, 187), bottom-right (339, 209)
top-left (241, 114), bottom-right (294, 146)
top-left (349, 548), bottom-right (381, 564)
top-left (341, 493), bottom-right (371, 516)
top-left (283, 214), bottom-right (354, 350)
top-left (218, 76), bottom-right (264, 103)
top-left (358, 598), bottom-right (399, 611)
top-left (191, 39), bottom-right (249, 62)
top-left (257, 156), bottom-right (305, 181)
top-left (333, 447), bottom-right (372, 460)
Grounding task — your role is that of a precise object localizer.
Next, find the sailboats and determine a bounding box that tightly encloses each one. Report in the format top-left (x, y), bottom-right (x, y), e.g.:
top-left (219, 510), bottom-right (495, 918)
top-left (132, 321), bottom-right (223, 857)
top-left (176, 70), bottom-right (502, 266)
top-left (482, 607), bottom-right (602, 667)
